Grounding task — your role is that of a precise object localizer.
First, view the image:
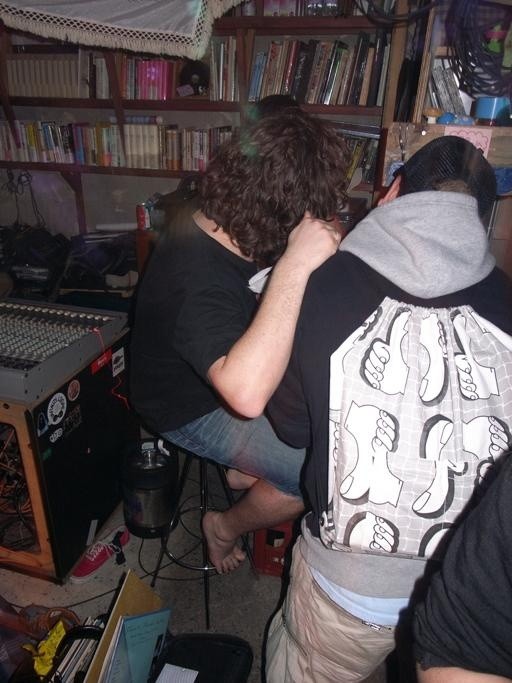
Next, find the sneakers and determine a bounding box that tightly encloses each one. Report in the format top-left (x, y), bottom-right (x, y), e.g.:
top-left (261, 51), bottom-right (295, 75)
top-left (71, 527), bottom-right (130, 585)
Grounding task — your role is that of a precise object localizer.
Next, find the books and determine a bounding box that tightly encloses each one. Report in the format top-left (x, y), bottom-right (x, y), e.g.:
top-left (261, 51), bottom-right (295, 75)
top-left (49, 564), bottom-right (171, 683)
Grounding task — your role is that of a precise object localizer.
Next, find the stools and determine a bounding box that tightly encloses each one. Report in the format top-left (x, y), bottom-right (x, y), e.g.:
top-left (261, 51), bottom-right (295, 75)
top-left (147, 443), bottom-right (260, 624)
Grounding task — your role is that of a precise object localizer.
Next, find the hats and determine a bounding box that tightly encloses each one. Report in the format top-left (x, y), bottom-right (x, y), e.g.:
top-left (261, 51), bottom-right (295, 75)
top-left (393, 137), bottom-right (495, 217)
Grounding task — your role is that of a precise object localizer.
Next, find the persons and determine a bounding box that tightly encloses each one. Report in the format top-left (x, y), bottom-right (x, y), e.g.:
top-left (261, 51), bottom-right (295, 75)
top-left (407, 452), bottom-right (512, 681)
top-left (261, 132), bottom-right (511, 682)
top-left (122, 107), bottom-right (354, 576)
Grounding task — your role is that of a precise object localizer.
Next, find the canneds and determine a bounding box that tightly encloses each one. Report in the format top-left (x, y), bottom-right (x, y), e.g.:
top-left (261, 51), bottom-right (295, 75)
top-left (136, 201), bottom-right (155, 231)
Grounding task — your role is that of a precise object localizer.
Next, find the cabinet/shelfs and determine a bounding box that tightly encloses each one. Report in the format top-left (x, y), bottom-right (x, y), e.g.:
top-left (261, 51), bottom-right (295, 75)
top-left (1, 0), bottom-right (246, 254)
top-left (385, 1), bottom-right (512, 277)
top-left (240, 1), bottom-right (412, 210)
